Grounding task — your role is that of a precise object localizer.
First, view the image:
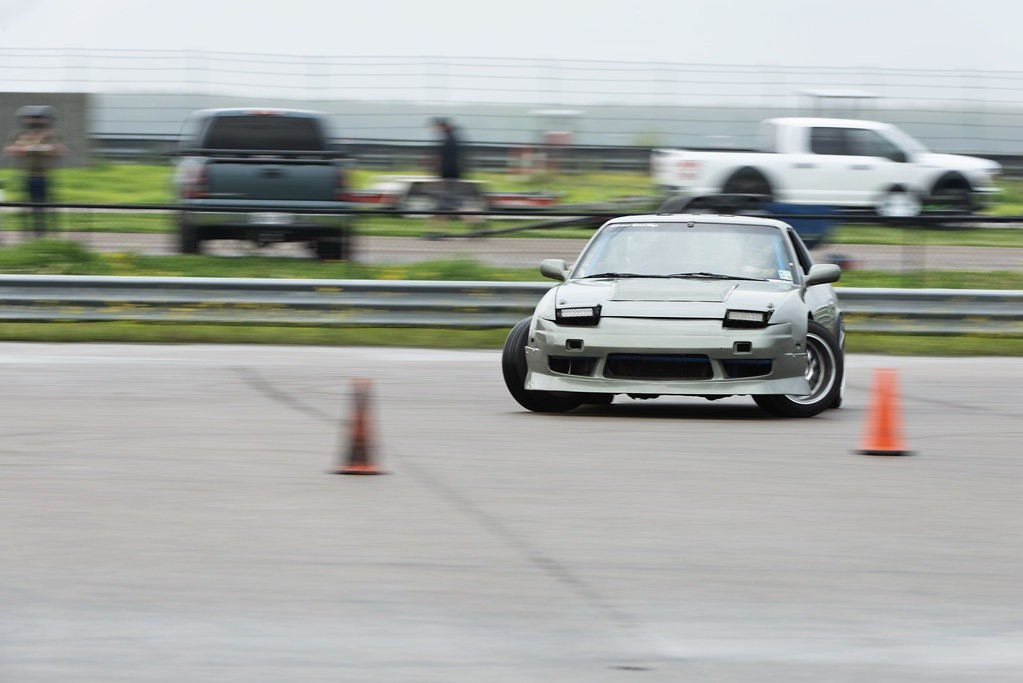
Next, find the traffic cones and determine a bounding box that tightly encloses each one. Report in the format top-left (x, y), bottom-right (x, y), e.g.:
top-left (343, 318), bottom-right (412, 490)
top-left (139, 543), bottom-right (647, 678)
top-left (328, 377), bottom-right (389, 474)
top-left (849, 365), bottom-right (911, 457)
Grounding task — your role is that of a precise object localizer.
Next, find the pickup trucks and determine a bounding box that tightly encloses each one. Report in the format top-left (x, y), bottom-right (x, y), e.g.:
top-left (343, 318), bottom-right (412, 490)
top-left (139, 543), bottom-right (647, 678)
top-left (167, 107), bottom-right (356, 262)
top-left (649, 115), bottom-right (1002, 229)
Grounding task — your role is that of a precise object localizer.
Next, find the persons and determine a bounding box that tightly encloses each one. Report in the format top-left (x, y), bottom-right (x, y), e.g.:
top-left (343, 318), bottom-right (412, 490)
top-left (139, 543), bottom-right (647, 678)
top-left (6, 105), bottom-right (59, 237)
top-left (725, 240), bottom-right (776, 278)
top-left (432, 117), bottom-right (463, 220)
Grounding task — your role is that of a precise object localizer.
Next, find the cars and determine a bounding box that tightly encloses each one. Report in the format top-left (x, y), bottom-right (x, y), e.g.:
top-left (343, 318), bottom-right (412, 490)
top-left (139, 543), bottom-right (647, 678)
top-left (501, 212), bottom-right (848, 421)
